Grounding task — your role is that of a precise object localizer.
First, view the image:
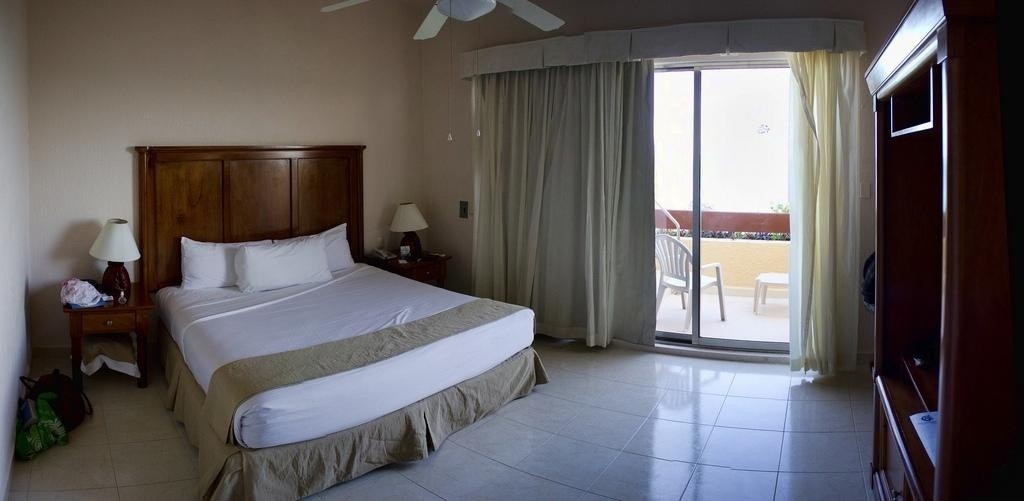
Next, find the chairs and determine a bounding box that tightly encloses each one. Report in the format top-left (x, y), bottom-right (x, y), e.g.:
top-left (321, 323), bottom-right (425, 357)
top-left (654, 233), bottom-right (726, 331)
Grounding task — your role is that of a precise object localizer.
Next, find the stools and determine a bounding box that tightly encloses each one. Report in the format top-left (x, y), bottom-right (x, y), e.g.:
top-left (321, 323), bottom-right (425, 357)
top-left (752, 272), bottom-right (790, 315)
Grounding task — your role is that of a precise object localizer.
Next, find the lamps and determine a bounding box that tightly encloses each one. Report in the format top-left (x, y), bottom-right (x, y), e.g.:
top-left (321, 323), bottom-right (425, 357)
top-left (89, 218), bottom-right (142, 297)
top-left (389, 201), bottom-right (428, 252)
top-left (436, 0), bottom-right (496, 21)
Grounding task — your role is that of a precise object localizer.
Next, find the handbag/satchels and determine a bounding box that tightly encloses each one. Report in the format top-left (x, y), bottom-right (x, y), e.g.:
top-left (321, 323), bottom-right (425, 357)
top-left (59, 277), bottom-right (99, 306)
top-left (15, 392), bottom-right (68, 460)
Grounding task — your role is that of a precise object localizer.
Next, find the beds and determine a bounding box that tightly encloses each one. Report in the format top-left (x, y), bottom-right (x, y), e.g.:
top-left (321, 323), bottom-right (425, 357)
top-left (149, 264), bottom-right (549, 501)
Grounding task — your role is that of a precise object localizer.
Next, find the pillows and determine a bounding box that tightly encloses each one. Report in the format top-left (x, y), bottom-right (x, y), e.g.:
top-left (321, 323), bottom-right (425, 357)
top-left (234, 234), bottom-right (332, 293)
top-left (273, 223), bottom-right (354, 271)
top-left (178, 236), bottom-right (272, 289)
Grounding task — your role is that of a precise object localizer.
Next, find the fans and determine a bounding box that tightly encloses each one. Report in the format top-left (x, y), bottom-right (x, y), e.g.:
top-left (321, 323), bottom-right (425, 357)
top-left (320, 0), bottom-right (567, 42)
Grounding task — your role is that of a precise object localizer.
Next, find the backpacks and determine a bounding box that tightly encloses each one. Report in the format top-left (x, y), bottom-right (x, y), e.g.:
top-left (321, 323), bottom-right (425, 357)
top-left (20, 368), bottom-right (92, 430)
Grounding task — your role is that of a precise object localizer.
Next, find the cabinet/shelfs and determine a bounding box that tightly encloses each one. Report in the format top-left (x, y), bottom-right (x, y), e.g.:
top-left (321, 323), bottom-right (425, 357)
top-left (861, 0), bottom-right (1023, 500)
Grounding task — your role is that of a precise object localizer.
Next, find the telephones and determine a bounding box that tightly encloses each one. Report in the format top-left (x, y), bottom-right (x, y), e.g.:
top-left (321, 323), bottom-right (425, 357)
top-left (374, 247), bottom-right (398, 260)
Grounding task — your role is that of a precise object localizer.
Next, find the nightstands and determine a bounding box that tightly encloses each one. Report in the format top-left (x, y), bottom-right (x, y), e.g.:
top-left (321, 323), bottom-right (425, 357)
top-left (59, 281), bottom-right (156, 388)
top-left (366, 250), bottom-right (451, 290)
